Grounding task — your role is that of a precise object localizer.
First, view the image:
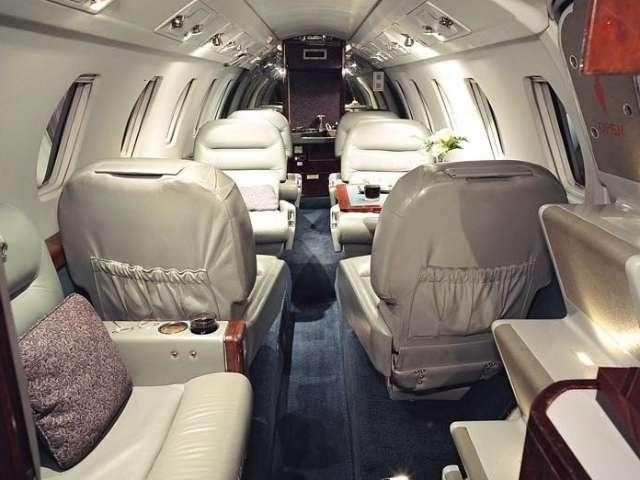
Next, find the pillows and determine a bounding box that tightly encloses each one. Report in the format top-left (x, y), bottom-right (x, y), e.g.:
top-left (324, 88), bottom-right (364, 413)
top-left (16, 295), bottom-right (131, 471)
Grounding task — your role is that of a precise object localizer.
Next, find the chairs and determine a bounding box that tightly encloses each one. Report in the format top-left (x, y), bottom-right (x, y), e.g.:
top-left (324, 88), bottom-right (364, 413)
top-left (59, 157), bottom-right (294, 369)
top-left (1, 202), bottom-right (251, 480)
top-left (329, 107), bottom-right (443, 253)
top-left (335, 160), bottom-right (567, 403)
top-left (190, 108), bottom-right (304, 259)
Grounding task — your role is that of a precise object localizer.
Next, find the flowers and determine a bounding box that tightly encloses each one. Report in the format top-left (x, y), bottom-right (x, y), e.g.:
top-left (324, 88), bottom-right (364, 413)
top-left (428, 126), bottom-right (467, 167)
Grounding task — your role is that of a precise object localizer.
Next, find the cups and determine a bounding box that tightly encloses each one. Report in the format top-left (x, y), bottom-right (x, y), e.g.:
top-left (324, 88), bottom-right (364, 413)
top-left (364, 184), bottom-right (381, 200)
top-left (189, 310), bottom-right (219, 335)
